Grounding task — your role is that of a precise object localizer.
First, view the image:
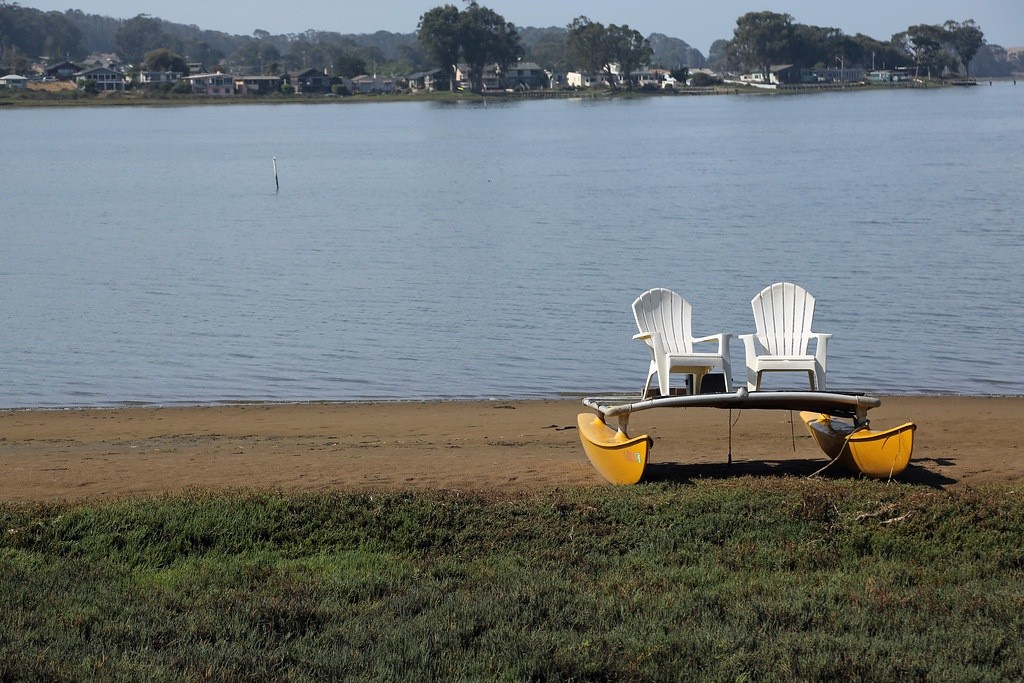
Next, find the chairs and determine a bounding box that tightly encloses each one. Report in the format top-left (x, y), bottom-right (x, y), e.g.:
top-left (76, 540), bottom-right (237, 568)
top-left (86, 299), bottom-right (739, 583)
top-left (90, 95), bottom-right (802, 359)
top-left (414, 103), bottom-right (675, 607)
top-left (738, 281), bottom-right (833, 392)
top-left (631, 287), bottom-right (734, 396)
